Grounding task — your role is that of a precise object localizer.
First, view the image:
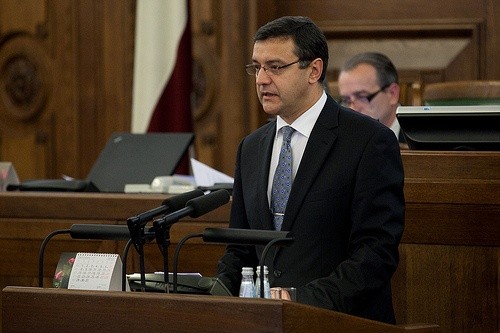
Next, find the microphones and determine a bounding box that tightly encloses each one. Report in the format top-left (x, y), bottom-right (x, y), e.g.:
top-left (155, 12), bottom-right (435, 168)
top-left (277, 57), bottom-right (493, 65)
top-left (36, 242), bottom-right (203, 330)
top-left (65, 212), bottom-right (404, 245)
top-left (152, 189), bottom-right (231, 228)
top-left (126, 188), bottom-right (204, 225)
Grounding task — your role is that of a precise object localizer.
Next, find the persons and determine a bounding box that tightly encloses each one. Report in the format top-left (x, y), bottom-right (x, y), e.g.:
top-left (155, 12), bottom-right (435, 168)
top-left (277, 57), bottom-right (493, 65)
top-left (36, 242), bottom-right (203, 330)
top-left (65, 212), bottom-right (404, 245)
top-left (338, 51), bottom-right (405, 144)
top-left (218, 16), bottom-right (406, 325)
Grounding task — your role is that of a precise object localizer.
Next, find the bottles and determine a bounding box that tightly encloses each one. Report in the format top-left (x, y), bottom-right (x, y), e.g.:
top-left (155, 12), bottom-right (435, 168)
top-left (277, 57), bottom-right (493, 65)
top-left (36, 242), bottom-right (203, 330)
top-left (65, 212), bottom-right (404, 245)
top-left (239, 267), bottom-right (256, 298)
top-left (254, 266), bottom-right (270, 298)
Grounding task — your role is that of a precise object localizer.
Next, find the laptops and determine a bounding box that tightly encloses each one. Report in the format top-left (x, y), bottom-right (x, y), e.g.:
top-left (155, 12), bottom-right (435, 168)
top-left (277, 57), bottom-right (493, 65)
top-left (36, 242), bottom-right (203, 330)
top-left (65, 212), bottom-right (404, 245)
top-left (86, 134), bottom-right (194, 193)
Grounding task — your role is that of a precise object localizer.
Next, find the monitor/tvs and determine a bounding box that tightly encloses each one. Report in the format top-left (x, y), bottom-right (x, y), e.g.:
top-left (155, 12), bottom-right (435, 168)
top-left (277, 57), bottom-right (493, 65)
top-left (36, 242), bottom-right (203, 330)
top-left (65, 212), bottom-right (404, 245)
top-left (125, 273), bottom-right (233, 296)
top-left (396, 106), bottom-right (500, 150)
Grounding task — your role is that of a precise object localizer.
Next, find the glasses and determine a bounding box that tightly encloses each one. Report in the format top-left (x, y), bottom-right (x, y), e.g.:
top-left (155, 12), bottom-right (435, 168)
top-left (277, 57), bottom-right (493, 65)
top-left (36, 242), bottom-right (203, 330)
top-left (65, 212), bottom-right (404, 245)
top-left (334, 84), bottom-right (391, 109)
top-left (245, 56), bottom-right (314, 76)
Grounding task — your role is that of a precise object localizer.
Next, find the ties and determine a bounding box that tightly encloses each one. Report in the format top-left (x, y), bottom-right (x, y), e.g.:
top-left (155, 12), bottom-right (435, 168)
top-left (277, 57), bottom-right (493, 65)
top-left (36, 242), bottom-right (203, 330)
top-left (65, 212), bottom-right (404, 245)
top-left (270, 126), bottom-right (296, 232)
